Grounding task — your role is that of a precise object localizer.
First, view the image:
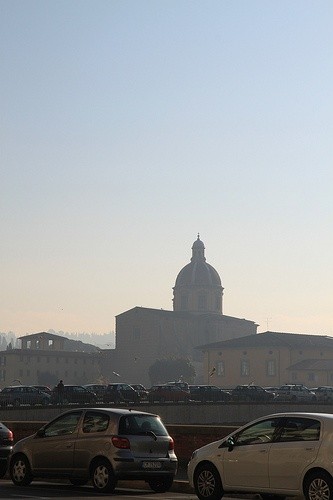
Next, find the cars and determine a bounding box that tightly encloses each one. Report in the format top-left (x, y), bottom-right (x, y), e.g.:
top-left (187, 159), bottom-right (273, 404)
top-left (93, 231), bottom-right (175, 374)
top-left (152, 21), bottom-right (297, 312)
top-left (0, 381), bottom-right (332, 407)
top-left (6, 408), bottom-right (177, 494)
top-left (186, 413), bottom-right (333, 500)
top-left (0, 421), bottom-right (14, 462)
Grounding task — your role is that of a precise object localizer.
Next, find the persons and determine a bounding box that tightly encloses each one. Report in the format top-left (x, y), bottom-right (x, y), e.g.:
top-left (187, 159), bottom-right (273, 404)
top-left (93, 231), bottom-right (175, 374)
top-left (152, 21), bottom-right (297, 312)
top-left (55, 380), bottom-right (65, 406)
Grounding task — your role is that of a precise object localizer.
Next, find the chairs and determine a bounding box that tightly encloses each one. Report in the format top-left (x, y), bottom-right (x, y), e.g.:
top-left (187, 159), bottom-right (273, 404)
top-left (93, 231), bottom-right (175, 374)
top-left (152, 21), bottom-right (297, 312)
top-left (284, 423), bottom-right (298, 441)
